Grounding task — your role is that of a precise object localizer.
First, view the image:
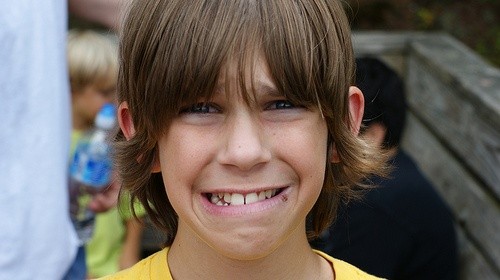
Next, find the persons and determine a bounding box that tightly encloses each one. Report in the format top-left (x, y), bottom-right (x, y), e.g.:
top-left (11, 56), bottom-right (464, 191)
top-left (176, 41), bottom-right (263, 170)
top-left (0, 0), bottom-right (459, 280)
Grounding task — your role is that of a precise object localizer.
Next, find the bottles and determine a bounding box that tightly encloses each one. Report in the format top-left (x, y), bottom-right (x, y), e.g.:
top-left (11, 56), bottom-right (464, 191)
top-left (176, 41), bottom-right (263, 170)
top-left (68, 104), bottom-right (118, 239)
